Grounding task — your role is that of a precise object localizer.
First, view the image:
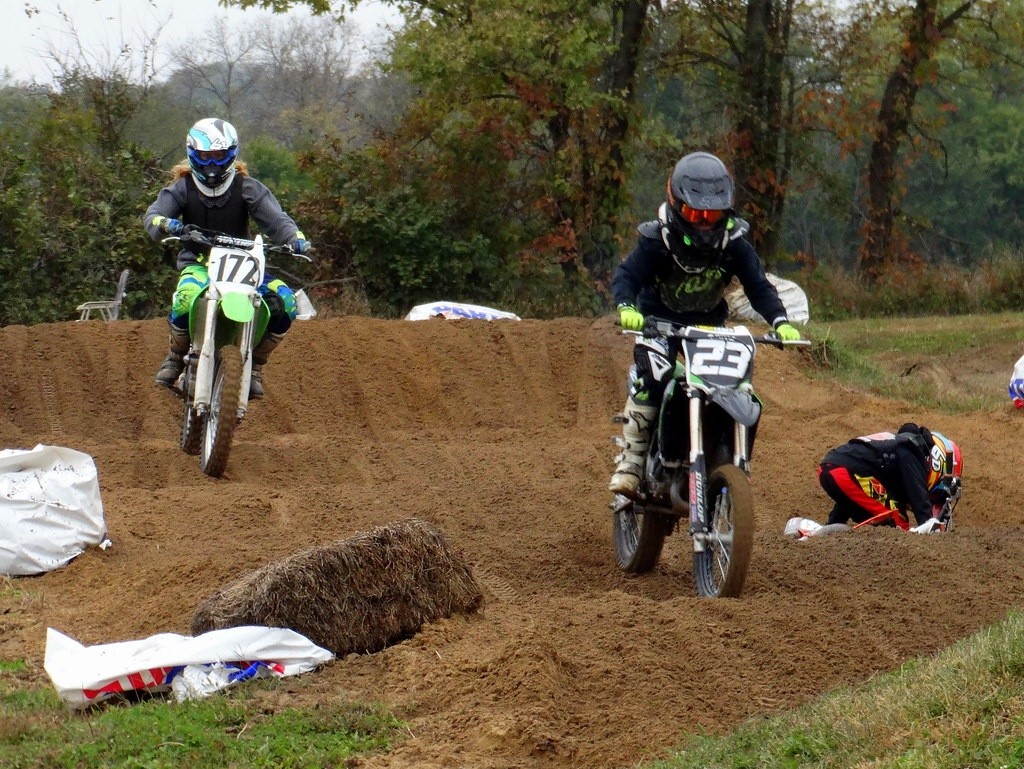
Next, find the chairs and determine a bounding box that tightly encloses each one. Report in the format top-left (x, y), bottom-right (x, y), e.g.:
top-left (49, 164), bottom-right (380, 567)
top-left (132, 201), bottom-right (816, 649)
top-left (77, 271), bottom-right (129, 322)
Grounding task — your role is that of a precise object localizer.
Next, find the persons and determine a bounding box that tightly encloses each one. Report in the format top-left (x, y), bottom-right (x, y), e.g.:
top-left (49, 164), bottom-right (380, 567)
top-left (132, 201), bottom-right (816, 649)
top-left (143, 118), bottom-right (310, 399)
top-left (609, 152), bottom-right (802, 495)
top-left (784, 422), bottom-right (944, 540)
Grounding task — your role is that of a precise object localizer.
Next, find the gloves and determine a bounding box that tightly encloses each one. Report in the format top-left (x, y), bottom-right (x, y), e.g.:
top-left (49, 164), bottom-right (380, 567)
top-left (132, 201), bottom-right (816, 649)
top-left (153, 215), bottom-right (184, 235)
top-left (290, 230), bottom-right (312, 256)
top-left (616, 302), bottom-right (644, 331)
top-left (773, 316), bottom-right (800, 341)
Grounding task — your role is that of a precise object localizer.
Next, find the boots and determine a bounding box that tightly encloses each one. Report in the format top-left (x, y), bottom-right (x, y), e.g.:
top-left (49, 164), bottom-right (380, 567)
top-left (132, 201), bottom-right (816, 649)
top-left (155, 319), bottom-right (191, 388)
top-left (609, 396), bottom-right (657, 495)
top-left (248, 328), bottom-right (287, 400)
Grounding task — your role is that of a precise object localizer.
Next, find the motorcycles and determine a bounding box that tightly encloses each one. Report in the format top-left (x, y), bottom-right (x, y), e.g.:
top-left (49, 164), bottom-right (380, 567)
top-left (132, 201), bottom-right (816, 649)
top-left (609, 314), bottom-right (812, 598)
top-left (161, 224), bottom-right (317, 476)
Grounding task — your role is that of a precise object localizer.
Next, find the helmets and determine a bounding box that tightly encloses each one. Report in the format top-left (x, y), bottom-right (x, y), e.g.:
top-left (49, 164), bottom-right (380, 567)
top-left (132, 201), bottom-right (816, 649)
top-left (927, 431), bottom-right (963, 492)
top-left (667, 152), bottom-right (737, 224)
top-left (186, 118), bottom-right (240, 189)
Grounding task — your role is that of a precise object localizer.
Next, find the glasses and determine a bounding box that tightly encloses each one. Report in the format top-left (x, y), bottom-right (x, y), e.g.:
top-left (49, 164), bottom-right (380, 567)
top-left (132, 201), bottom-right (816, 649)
top-left (187, 145), bottom-right (238, 166)
top-left (667, 177), bottom-right (727, 225)
